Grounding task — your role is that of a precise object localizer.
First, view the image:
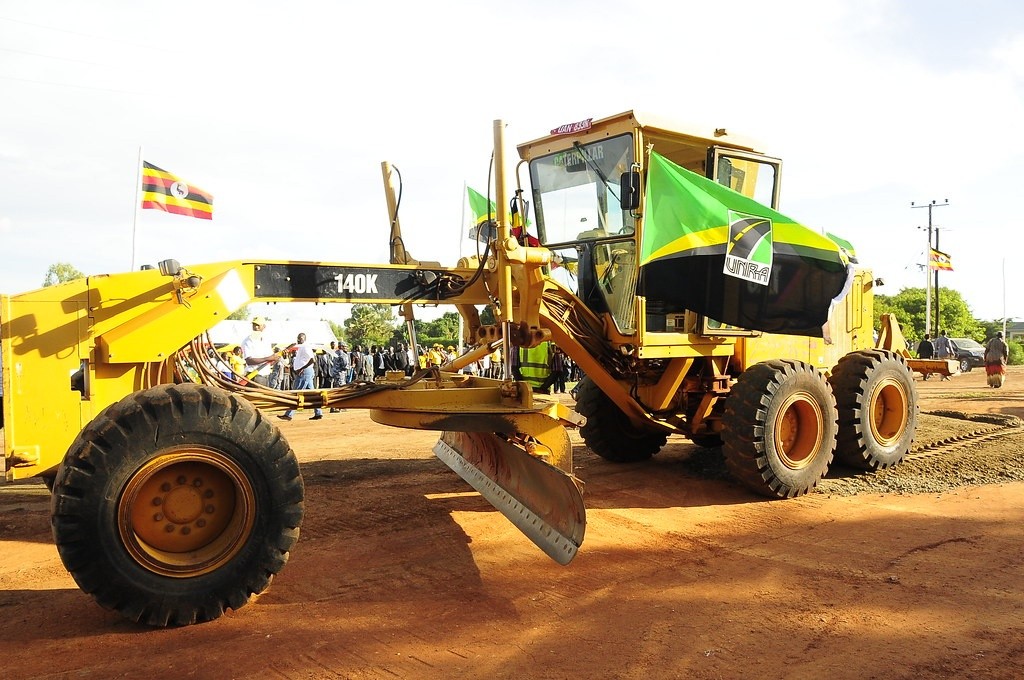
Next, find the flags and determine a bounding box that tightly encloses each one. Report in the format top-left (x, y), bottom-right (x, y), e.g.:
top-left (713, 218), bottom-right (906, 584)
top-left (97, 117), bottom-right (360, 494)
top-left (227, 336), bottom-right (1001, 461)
top-left (636, 152), bottom-right (861, 339)
top-left (466, 186), bottom-right (532, 246)
top-left (138, 160), bottom-right (214, 221)
top-left (930, 248), bottom-right (954, 271)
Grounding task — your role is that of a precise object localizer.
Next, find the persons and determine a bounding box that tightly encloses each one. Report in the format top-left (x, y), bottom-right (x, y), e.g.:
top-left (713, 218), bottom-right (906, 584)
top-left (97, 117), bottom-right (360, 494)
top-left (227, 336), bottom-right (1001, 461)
top-left (914, 334), bottom-right (935, 382)
top-left (242, 317), bottom-right (277, 396)
top-left (203, 342), bottom-right (580, 413)
top-left (277, 334), bottom-right (323, 420)
top-left (898, 323), bottom-right (909, 349)
top-left (873, 327), bottom-right (879, 343)
top-left (984, 332), bottom-right (1008, 387)
top-left (935, 331), bottom-right (955, 381)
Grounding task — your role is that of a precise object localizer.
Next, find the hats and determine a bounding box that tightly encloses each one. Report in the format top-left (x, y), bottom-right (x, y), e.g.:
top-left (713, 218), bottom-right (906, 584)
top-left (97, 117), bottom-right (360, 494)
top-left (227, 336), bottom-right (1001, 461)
top-left (940, 330), bottom-right (946, 335)
top-left (372, 345), bottom-right (377, 348)
top-left (338, 341), bottom-right (348, 347)
top-left (996, 332), bottom-right (1003, 337)
top-left (925, 334), bottom-right (929, 339)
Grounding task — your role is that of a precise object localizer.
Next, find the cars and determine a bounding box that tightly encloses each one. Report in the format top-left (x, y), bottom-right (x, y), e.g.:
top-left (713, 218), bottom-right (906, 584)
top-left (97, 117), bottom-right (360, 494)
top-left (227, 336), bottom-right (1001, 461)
top-left (931, 336), bottom-right (987, 372)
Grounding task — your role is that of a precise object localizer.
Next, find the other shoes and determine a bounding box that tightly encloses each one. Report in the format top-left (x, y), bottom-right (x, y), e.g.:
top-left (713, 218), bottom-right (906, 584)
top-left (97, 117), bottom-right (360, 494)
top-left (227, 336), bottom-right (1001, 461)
top-left (945, 376), bottom-right (951, 381)
top-left (923, 379), bottom-right (927, 380)
top-left (277, 414), bottom-right (292, 421)
top-left (309, 415), bottom-right (323, 420)
top-left (941, 379), bottom-right (943, 381)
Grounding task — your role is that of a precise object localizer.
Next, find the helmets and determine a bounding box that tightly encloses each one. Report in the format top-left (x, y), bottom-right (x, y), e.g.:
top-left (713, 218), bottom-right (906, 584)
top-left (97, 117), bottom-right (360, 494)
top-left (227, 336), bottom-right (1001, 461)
top-left (315, 349), bottom-right (324, 354)
top-left (440, 344), bottom-right (444, 348)
top-left (433, 343), bottom-right (439, 347)
top-left (448, 346), bottom-right (455, 350)
top-left (251, 316), bottom-right (266, 331)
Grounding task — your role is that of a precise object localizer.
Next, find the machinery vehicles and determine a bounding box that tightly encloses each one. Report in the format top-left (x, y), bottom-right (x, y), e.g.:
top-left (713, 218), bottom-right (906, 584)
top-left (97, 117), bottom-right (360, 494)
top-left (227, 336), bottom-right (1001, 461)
top-left (0, 108), bottom-right (961, 630)
